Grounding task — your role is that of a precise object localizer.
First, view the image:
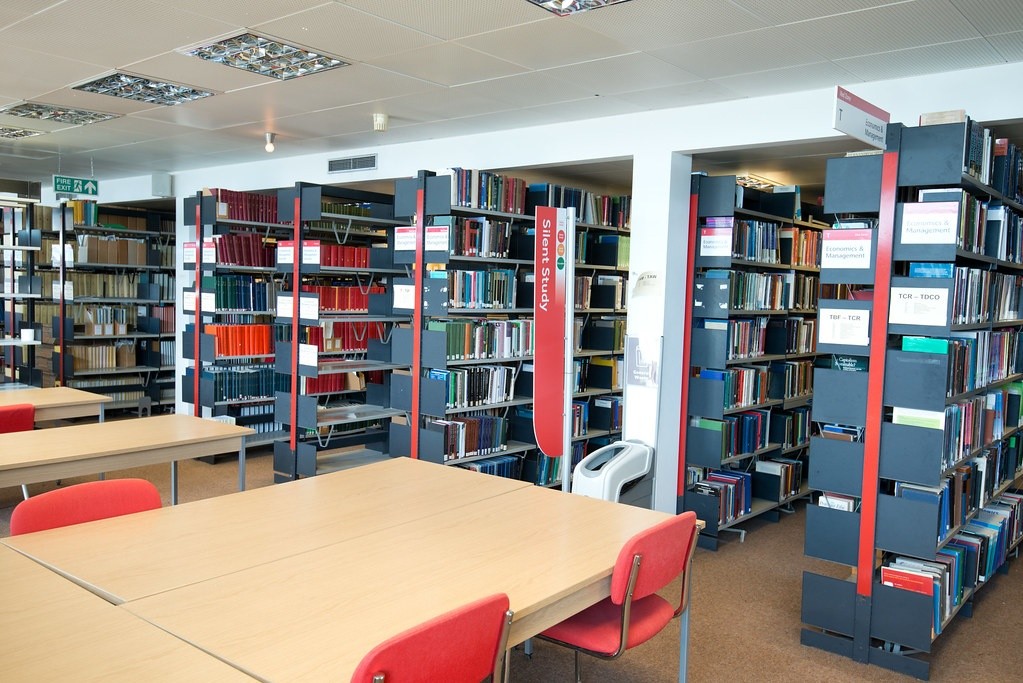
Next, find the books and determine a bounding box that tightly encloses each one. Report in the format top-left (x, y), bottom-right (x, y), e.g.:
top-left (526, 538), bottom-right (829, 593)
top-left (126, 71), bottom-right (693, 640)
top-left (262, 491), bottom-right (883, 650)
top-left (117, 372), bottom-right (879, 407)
top-left (412, 163), bottom-right (632, 487)
top-left (814, 109), bottom-right (1023, 640)
top-left (186, 186), bottom-right (386, 434)
top-left (0, 203), bottom-right (176, 401)
top-left (688, 173), bottom-right (823, 528)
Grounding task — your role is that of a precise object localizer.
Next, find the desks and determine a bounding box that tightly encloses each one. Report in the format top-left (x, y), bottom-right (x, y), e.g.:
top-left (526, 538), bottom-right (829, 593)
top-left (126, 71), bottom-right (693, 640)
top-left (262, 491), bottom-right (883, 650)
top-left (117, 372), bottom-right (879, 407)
top-left (0, 386), bottom-right (707, 683)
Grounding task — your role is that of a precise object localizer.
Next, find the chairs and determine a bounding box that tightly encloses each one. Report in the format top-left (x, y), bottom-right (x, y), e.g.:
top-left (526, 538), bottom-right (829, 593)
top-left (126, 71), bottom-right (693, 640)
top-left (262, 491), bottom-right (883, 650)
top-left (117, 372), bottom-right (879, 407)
top-left (351, 592), bottom-right (514, 683)
top-left (0, 403), bottom-right (36, 434)
top-left (503, 511), bottom-right (699, 683)
top-left (9, 477), bottom-right (163, 536)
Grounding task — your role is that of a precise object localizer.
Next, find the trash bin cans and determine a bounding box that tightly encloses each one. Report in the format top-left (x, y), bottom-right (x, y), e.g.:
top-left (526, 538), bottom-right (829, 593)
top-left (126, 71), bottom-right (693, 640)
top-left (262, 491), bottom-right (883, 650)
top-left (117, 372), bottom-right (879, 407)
top-left (571, 439), bottom-right (655, 511)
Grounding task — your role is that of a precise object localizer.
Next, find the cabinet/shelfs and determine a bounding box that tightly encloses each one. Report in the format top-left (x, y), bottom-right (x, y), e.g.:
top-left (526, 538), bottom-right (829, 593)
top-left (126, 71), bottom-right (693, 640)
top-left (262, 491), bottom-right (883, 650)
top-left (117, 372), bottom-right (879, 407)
top-left (272, 180), bottom-right (412, 488)
top-left (389, 169), bottom-right (632, 489)
top-left (799, 118), bottom-right (1023, 683)
top-left (182, 186), bottom-right (386, 463)
top-left (0, 196), bottom-right (176, 418)
top-left (675, 172), bottom-right (851, 551)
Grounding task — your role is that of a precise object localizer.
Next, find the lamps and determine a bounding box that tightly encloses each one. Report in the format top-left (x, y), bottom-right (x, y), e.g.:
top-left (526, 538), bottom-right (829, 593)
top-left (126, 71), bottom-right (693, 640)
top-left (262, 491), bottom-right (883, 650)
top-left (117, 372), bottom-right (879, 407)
top-left (263, 133), bottom-right (277, 153)
